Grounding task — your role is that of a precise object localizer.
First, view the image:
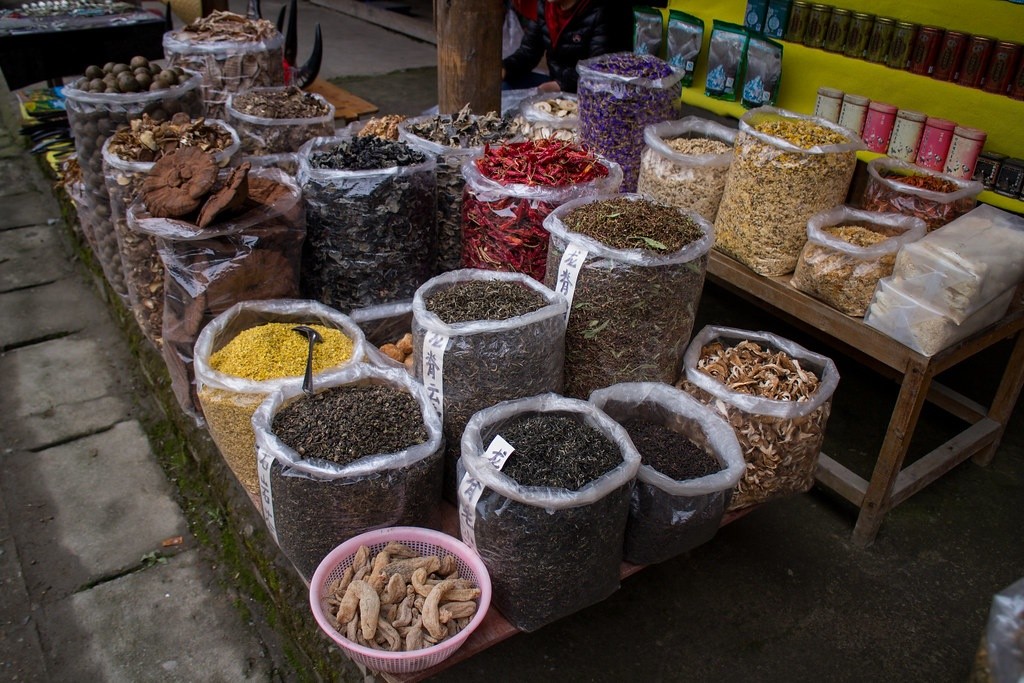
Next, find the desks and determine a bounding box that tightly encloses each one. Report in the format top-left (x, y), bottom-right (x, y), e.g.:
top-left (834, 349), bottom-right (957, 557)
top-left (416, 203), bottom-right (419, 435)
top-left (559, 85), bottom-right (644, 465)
top-left (303, 74), bottom-right (379, 128)
top-left (706, 249), bottom-right (1024, 551)
top-left (0, 5), bottom-right (169, 91)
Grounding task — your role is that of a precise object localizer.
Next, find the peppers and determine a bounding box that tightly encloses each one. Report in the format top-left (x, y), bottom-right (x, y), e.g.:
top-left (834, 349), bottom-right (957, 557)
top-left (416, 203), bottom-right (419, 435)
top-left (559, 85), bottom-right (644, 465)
top-left (863, 171), bottom-right (967, 233)
top-left (458, 132), bottom-right (610, 281)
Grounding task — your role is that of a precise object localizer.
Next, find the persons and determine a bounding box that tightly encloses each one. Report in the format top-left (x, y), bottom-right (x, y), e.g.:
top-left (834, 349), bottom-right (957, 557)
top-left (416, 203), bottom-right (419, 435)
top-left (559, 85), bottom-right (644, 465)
top-left (501, 0), bottom-right (633, 94)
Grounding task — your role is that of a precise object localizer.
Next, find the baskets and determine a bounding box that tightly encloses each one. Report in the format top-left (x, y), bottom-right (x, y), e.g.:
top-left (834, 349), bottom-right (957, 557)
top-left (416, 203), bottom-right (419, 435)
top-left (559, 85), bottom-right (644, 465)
top-left (309, 525), bottom-right (492, 674)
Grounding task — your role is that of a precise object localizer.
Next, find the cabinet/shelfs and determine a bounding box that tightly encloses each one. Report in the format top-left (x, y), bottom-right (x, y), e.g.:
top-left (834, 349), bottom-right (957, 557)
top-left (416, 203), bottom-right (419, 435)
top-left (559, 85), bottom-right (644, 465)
top-left (650, 1), bottom-right (1024, 220)
